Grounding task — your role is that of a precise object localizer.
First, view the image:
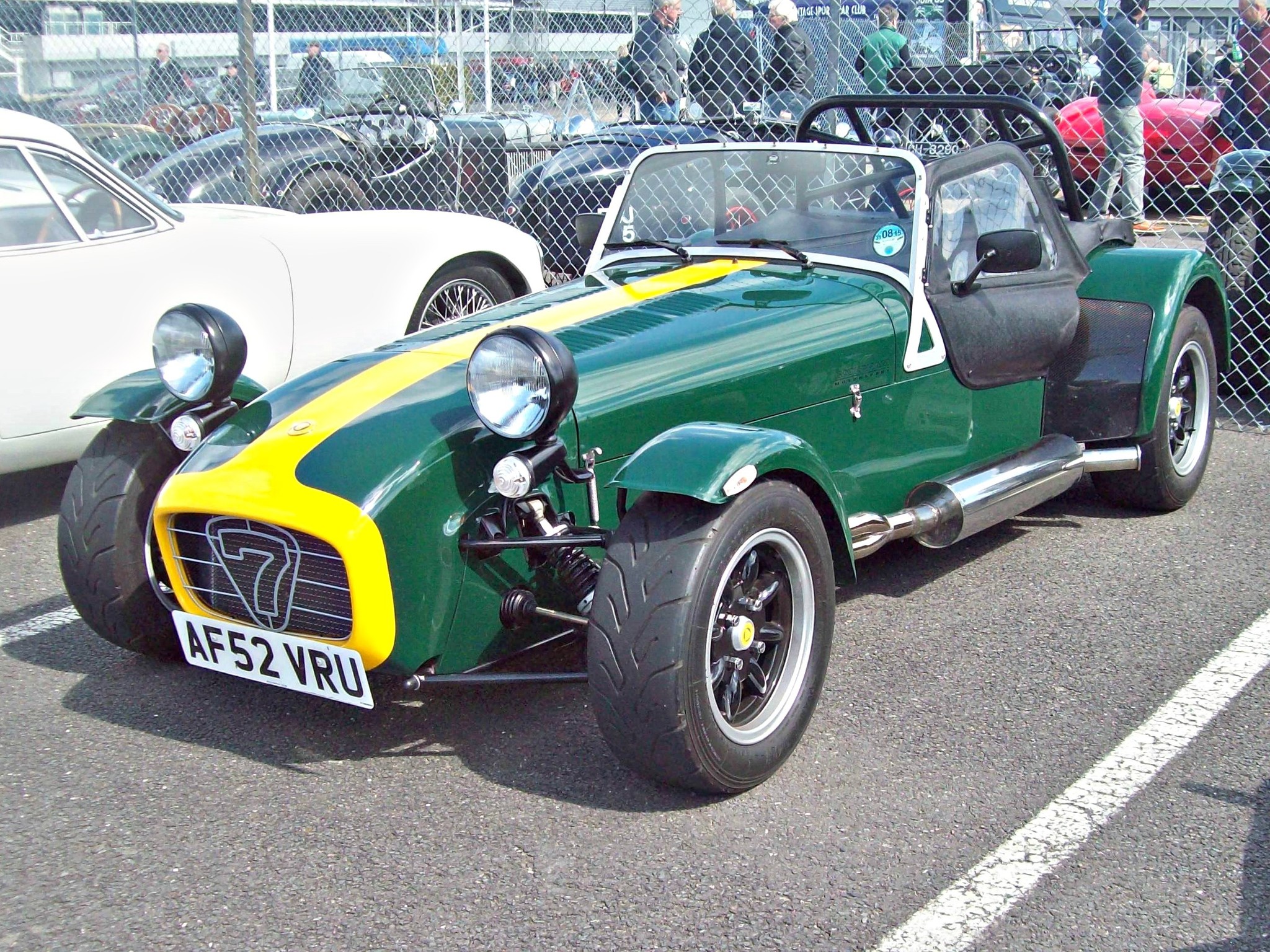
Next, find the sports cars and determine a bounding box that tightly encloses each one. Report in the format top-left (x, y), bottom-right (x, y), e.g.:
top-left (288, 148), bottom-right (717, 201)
top-left (1052, 84), bottom-right (1235, 207)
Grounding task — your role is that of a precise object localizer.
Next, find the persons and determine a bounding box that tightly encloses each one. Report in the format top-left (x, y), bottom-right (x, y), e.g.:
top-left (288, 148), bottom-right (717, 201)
top-left (216, 50), bottom-right (270, 111)
top-left (1078, 0), bottom-right (1270, 231)
top-left (491, 0), bottom-right (813, 144)
top-left (855, 4), bottom-right (911, 133)
top-left (146, 42), bottom-right (184, 105)
top-left (292, 40), bottom-right (336, 108)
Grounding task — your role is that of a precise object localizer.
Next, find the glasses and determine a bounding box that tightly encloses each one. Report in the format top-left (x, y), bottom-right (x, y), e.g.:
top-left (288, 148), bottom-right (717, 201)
top-left (767, 12), bottom-right (787, 18)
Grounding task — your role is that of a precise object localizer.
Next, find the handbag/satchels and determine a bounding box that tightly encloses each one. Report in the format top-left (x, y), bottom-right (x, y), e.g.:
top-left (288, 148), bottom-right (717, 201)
top-left (616, 41), bottom-right (637, 89)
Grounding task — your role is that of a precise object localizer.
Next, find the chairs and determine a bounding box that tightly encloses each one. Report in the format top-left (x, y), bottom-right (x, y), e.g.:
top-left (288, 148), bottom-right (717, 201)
top-left (765, 91), bottom-right (816, 122)
top-left (691, 90), bottom-right (732, 116)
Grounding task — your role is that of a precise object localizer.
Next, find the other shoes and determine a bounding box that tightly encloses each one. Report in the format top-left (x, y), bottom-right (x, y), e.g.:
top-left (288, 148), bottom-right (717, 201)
top-left (1133, 220), bottom-right (1166, 234)
top-left (1096, 214), bottom-right (1114, 219)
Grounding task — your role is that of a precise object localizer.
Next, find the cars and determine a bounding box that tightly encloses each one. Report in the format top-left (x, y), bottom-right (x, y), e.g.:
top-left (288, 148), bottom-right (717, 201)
top-left (1, 39), bottom-right (1105, 285)
top-left (1201, 149), bottom-right (1270, 401)
top-left (1, 109), bottom-right (549, 479)
top-left (56, 94), bottom-right (1234, 794)
